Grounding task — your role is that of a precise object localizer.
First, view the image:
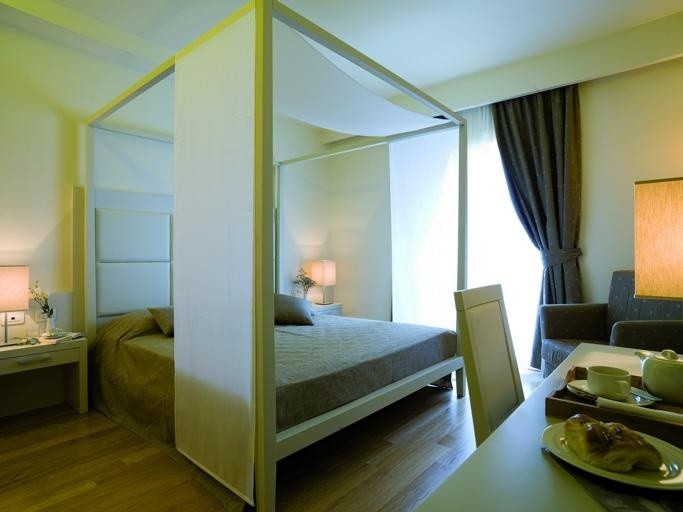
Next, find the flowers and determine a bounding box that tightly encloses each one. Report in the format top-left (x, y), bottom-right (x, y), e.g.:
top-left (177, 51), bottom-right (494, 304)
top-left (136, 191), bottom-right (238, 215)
top-left (27, 280), bottom-right (59, 318)
top-left (291, 269), bottom-right (316, 292)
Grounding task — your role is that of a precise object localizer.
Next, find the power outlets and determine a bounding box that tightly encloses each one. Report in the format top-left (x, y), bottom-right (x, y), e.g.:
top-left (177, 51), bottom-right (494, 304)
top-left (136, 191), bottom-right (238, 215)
top-left (34, 309), bottom-right (57, 321)
top-left (0, 312), bottom-right (24, 325)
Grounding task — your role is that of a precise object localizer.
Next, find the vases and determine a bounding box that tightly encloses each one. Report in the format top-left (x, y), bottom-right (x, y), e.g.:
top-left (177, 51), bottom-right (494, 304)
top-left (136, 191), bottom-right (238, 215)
top-left (42, 319), bottom-right (63, 337)
top-left (302, 294), bottom-right (311, 304)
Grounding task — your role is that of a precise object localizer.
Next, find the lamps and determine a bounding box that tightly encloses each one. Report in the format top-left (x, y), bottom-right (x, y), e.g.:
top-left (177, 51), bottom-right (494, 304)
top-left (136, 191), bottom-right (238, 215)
top-left (311, 260), bottom-right (337, 305)
top-left (632, 176), bottom-right (683, 302)
top-left (0, 266), bottom-right (30, 346)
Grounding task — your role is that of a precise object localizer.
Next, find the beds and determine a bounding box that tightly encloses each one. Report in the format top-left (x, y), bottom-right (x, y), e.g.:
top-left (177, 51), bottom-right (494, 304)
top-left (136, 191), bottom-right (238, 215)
top-left (78, 189), bottom-right (468, 510)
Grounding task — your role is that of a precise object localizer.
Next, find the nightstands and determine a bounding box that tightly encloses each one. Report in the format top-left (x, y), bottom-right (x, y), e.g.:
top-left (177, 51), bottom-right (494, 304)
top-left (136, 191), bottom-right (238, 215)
top-left (0, 335), bottom-right (87, 415)
top-left (310, 300), bottom-right (342, 317)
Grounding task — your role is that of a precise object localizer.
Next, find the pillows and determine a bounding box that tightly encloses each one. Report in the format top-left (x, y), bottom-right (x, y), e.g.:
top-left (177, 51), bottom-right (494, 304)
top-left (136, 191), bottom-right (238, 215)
top-left (273, 291), bottom-right (314, 325)
top-left (146, 305), bottom-right (174, 335)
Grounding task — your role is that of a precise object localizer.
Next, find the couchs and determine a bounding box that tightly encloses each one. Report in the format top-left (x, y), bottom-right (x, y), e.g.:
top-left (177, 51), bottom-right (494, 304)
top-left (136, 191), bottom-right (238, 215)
top-left (538, 270), bottom-right (682, 379)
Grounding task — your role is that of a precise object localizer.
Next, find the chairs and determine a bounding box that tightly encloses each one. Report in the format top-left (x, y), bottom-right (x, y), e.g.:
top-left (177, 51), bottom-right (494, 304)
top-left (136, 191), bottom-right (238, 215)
top-left (452, 282), bottom-right (529, 448)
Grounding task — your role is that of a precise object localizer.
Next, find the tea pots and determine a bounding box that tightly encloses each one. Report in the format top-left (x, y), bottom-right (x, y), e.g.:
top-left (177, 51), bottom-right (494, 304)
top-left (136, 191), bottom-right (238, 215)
top-left (634, 347), bottom-right (683, 406)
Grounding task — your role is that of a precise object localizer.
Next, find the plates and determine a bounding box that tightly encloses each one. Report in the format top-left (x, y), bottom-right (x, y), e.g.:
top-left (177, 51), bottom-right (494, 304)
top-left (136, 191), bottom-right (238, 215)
top-left (542, 422), bottom-right (683, 490)
top-left (566, 378), bottom-right (653, 407)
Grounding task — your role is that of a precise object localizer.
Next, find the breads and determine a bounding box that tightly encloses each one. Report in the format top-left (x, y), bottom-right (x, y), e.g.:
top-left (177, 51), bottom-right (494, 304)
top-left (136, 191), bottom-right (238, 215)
top-left (565, 415), bottom-right (664, 472)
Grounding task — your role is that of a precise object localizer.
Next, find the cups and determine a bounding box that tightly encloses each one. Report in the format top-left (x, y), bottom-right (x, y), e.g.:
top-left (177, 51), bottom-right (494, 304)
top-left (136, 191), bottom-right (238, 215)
top-left (586, 365), bottom-right (632, 403)
